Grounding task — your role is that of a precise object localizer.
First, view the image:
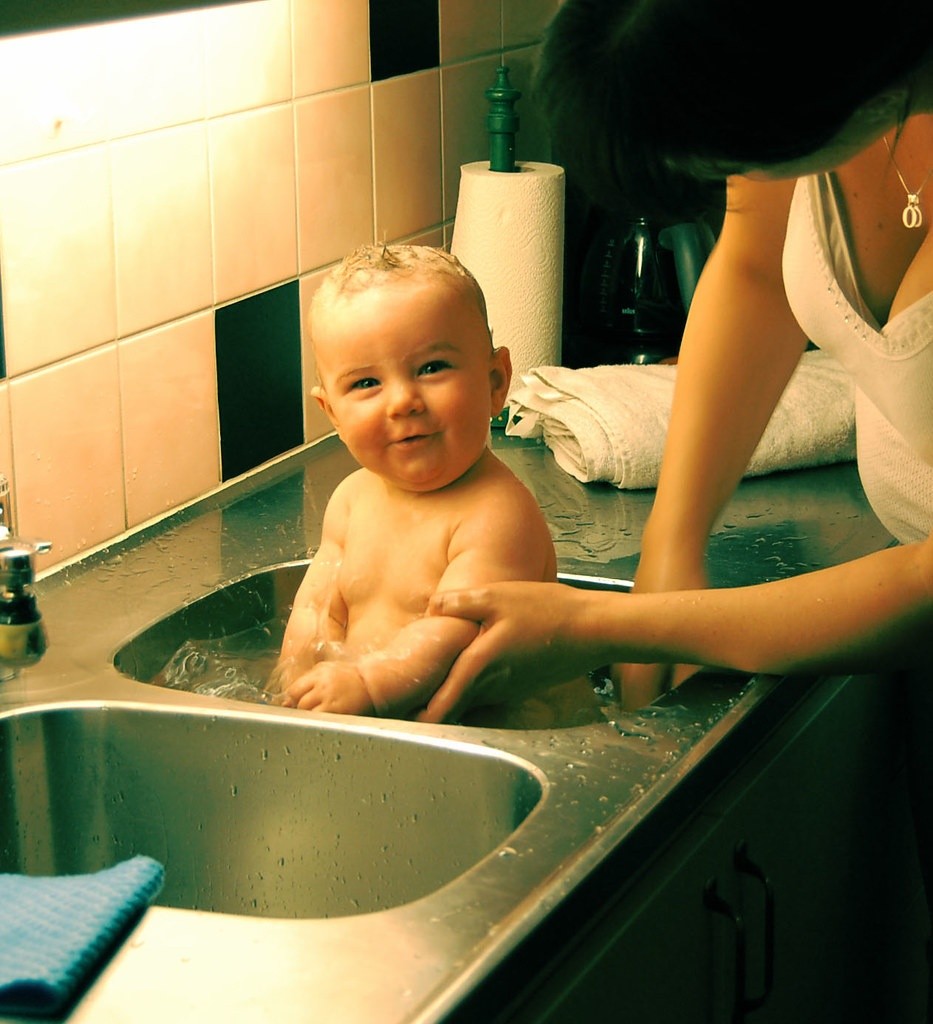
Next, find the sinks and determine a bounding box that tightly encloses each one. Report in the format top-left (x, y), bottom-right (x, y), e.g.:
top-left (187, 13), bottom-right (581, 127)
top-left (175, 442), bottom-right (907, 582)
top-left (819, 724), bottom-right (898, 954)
top-left (109, 553), bottom-right (705, 732)
top-left (0, 702), bottom-right (549, 925)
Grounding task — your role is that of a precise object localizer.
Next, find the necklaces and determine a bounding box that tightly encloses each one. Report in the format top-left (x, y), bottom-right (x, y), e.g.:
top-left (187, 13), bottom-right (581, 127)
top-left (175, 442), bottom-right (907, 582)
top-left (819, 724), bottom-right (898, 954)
top-left (884, 139), bottom-right (933, 229)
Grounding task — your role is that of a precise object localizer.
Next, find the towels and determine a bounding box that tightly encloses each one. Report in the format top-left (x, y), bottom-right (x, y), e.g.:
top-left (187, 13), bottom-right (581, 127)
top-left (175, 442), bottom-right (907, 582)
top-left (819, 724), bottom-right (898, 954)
top-left (0, 852), bottom-right (170, 1022)
top-left (504, 349), bottom-right (855, 491)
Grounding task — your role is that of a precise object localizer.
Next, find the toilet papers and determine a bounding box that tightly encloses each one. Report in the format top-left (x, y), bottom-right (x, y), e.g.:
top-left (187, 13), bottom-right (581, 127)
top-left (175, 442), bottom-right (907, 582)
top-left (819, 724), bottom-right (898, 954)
top-left (451, 158), bottom-right (567, 409)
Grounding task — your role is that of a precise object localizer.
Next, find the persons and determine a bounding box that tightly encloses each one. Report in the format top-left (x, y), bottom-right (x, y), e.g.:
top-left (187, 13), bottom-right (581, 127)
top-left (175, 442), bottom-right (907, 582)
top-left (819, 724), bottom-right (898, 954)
top-left (261, 245), bottom-right (557, 713)
top-left (414, 0), bottom-right (933, 724)
